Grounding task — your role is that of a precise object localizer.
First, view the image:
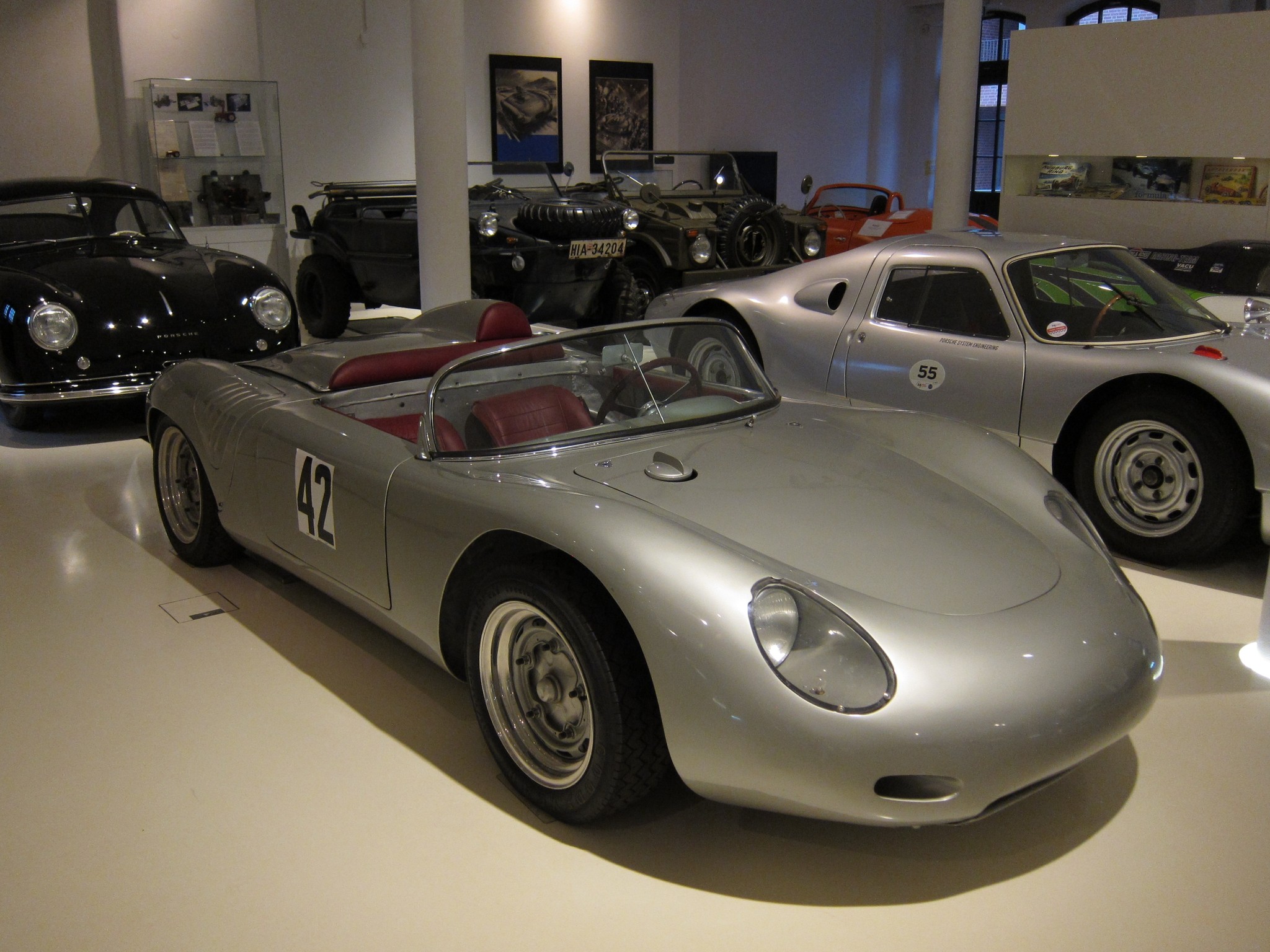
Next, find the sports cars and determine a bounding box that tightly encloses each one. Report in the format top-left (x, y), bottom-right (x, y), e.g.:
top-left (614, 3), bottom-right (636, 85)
top-left (782, 180), bottom-right (998, 273)
top-left (1022, 236), bottom-right (1270, 329)
top-left (285, 150), bottom-right (829, 342)
top-left (638, 226), bottom-right (1270, 586)
top-left (144, 296), bottom-right (1165, 827)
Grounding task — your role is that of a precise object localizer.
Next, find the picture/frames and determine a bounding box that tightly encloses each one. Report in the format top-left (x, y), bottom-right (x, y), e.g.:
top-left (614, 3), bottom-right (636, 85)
top-left (489, 54), bottom-right (563, 174)
top-left (589, 60), bottom-right (653, 173)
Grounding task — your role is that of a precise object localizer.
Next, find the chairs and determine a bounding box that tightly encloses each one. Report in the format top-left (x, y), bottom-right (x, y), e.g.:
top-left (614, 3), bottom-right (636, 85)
top-left (362, 413), bottom-right (467, 452)
top-left (467, 385), bottom-right (592, 447)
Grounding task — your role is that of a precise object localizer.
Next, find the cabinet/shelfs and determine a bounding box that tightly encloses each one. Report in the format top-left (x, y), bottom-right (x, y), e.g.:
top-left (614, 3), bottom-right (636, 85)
top-left (134, 79), bottom-right (291, 295)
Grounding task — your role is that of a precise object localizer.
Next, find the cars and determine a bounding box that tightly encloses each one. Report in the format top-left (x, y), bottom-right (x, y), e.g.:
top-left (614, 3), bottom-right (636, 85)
top-left (1, 173), bottom-right (302, 427)
